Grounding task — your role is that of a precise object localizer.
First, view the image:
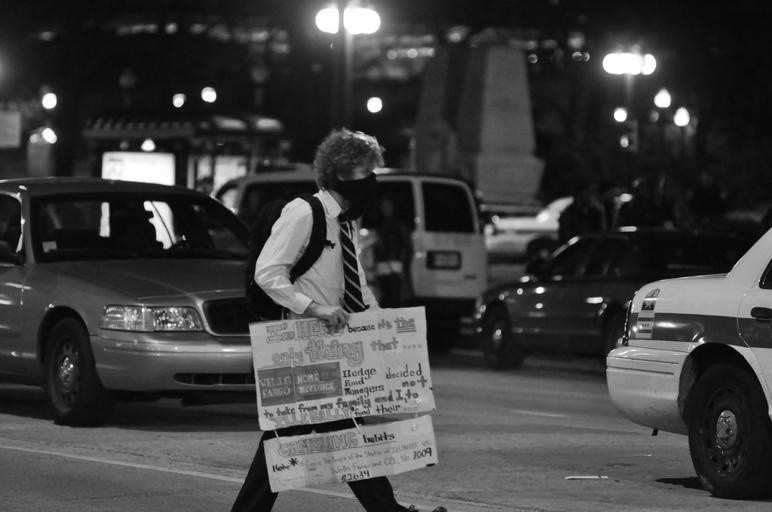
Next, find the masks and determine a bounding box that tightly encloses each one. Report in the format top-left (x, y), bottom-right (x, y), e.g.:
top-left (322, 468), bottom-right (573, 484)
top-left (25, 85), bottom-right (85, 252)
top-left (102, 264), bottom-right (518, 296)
top-left (338, 171), bottom-right (377, 215)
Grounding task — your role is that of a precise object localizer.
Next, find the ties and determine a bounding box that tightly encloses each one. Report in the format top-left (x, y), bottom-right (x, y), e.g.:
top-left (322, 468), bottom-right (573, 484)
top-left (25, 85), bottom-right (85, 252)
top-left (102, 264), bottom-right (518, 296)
top-left (337, 212), bottom-right (365, 314)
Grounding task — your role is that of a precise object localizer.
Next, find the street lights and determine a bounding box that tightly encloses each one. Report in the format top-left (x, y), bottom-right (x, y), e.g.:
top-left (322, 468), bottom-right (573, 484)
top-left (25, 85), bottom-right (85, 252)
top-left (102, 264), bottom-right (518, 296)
top-left (600, 46), bottom-right (658, 192)
top-left (315, 1), bottom-right (380, 134)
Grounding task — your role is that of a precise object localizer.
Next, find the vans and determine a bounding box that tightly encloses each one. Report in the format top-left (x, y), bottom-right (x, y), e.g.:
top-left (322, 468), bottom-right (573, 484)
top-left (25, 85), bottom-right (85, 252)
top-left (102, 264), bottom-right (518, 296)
top-left (209, 173), bottom-right (484, 352)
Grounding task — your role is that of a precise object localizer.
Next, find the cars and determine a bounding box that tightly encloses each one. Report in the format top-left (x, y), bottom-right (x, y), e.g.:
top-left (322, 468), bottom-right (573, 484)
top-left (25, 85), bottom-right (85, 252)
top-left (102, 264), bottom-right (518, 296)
top-left (607, 228), bottom-right (772, 497)
top-left (0, 177), bottom-right (289, 426)
top-left (470, 229), bottom-right (760, 371)
top-left (483, 195), bottom-right (638, 273)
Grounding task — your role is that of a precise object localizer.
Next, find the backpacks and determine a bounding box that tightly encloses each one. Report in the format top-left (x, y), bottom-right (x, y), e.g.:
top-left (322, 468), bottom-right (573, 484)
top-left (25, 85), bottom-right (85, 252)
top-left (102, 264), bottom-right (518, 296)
top-left (245, 192), bottom-right (336, 314)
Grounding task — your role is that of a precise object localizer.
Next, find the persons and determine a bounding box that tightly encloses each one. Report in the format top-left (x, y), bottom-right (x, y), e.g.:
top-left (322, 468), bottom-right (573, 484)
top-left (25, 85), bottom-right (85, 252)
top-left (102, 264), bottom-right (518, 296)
top-left (195, 176), bottom-right (215, 217)
top-left (559, 168), bottom-right (726, 281)
top-left (230, 125), bottom-right (449, 512)
